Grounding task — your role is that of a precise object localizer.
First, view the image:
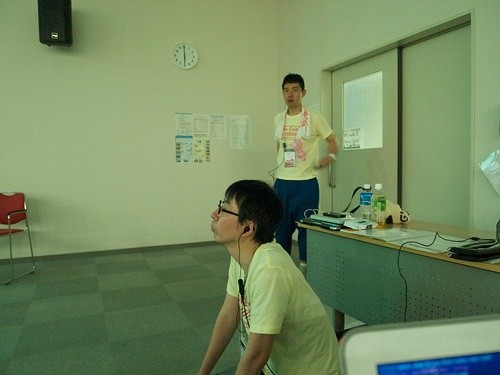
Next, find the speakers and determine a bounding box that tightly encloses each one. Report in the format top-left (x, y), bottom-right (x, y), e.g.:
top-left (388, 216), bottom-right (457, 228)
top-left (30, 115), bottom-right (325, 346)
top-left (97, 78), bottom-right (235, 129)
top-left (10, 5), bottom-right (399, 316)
top-left (38, 0), bottom-right (73, 45)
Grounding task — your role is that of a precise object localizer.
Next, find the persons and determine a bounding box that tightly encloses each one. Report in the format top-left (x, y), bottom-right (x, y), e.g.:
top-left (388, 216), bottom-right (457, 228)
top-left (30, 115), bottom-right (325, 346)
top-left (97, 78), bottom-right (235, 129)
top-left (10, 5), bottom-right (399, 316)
top-left (273, 72), bottom-right (338, 274)
top-left (197, 180), bottom-right (340, 375)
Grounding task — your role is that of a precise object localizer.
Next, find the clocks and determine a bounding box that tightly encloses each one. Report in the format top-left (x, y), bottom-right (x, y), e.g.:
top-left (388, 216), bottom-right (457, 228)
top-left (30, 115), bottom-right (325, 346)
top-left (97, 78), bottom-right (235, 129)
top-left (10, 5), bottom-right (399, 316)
top-left (172, 42), bottom-right (198, 70)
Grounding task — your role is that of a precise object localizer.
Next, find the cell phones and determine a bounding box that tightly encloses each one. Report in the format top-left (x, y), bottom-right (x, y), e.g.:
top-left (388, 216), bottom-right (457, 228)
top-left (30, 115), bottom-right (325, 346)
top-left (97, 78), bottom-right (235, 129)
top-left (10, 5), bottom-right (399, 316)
top-left (323, 212), bottom-right (346, 217)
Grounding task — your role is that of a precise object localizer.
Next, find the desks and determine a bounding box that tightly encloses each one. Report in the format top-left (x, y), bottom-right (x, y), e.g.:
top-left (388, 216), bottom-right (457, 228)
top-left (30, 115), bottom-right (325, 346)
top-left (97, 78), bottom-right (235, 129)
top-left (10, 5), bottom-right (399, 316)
top-left (297, 220), bottom-right (500, 341)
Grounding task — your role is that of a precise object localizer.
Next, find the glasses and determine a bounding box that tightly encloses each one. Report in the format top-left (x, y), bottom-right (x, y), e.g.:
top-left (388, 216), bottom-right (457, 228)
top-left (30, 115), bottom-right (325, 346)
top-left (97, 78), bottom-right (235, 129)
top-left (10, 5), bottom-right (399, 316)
top-left (217, 199), bottom-right (241, 218)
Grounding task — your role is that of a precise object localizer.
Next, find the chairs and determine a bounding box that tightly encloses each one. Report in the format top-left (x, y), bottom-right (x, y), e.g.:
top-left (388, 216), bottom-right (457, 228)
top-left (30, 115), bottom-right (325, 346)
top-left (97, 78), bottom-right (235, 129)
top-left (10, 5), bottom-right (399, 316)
top-left (0, 192), bottom-right (36, 284)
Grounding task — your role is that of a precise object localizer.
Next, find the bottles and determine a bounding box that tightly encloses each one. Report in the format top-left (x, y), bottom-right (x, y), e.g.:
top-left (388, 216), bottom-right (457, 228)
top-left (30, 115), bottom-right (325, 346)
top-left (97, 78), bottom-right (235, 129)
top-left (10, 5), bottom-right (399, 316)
top-left (359, 183), bottom-right (373, 219)
top-left (370, 184), bottom-right (386, 228)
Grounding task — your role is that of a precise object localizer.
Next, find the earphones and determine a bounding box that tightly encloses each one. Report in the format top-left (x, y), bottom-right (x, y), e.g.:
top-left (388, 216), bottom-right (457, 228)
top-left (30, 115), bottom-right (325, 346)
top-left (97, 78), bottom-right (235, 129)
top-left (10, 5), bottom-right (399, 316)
top-left (244, 226), bottom-right (250, 232)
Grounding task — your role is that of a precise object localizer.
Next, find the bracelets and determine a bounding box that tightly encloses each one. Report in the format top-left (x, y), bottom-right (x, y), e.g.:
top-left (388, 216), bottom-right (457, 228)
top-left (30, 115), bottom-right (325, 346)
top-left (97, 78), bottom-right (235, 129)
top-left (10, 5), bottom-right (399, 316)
top-left (328, 153), bottom-right (336, 160)
